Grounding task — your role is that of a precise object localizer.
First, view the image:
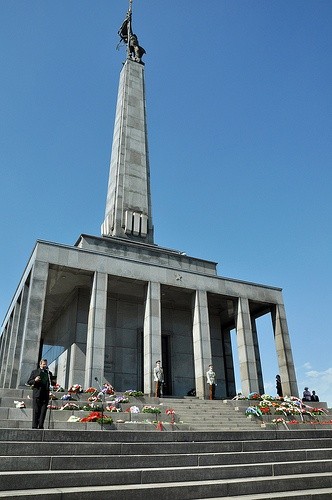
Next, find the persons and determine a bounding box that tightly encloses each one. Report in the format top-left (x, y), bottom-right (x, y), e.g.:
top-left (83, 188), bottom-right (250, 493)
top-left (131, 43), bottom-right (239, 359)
top-left (206, 364), bottom-right (218, 400)
top-left (126, 34), bottom-right (146, 62)
top-left (276, 375), bottom-right (283, 397)
top-left (154, 360), bottom-right (164, 398)
top-left (303, 387), bottom-right (319, 402)
top-left (27, 358), bottom-right (56, 429)
